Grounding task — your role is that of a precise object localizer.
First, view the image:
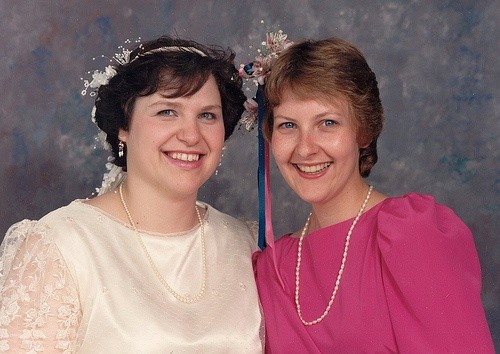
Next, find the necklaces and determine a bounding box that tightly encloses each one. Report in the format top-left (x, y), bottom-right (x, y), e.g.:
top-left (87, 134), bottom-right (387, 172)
top-left (295, 185), bottom-right (375, 325)
top-left (119, 182), bottom-right (206, 303)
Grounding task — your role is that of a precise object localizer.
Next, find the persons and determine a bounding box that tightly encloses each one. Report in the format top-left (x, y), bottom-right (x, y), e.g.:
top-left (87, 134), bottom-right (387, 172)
top-left (239, 38), bottom-right (495, 354)
top-left (0, 38), bottom-right (265, 354)
